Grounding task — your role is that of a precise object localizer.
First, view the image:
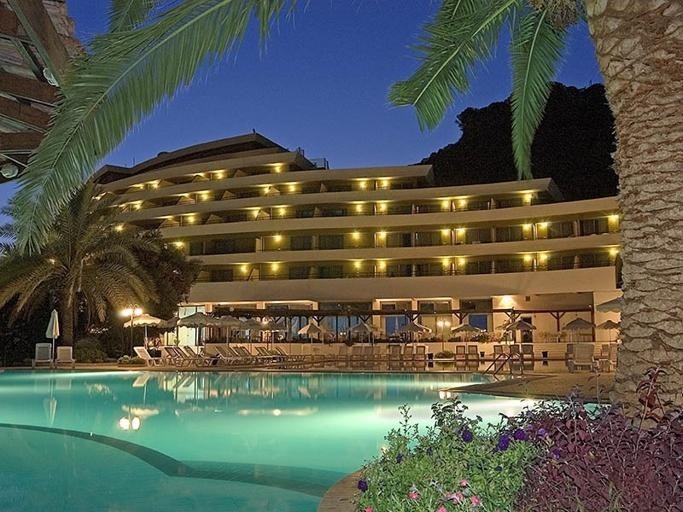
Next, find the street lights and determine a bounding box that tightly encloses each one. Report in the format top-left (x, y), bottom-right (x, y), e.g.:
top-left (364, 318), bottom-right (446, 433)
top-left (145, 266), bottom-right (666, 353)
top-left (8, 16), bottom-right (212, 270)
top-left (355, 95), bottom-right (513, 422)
top-left (120, 304), bottom-right (143, 357)
top-left (437, 319), bottom-right (451, 351)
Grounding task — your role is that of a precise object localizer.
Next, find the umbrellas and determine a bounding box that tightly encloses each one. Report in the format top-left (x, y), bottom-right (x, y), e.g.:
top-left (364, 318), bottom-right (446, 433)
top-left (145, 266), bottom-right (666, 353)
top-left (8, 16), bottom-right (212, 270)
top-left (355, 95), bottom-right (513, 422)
top-left (595, 296), bottom-right (623, 313)
top-left (44, 309), bottom-right (60, 361)
top-left (40, 379), bottom-right (59, 427)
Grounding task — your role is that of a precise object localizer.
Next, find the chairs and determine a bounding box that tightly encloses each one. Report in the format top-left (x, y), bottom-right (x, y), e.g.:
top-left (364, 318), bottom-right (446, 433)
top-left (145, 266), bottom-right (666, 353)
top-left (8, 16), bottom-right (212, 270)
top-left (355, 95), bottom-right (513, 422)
top-left (32, 343), bottom-right (53, 369)
top-left (347, 345), bottom-right (427, 366)
top-left (493, 345), bottom-right (534, 366)
top-left (456, 346), bottom-right (479, 366)
top-left (55, 346), bottom-right (75, 369)
top-left (565, 344), bottom-right (611, 372)
top-left (134, 345), bottom-right (350, 367)
